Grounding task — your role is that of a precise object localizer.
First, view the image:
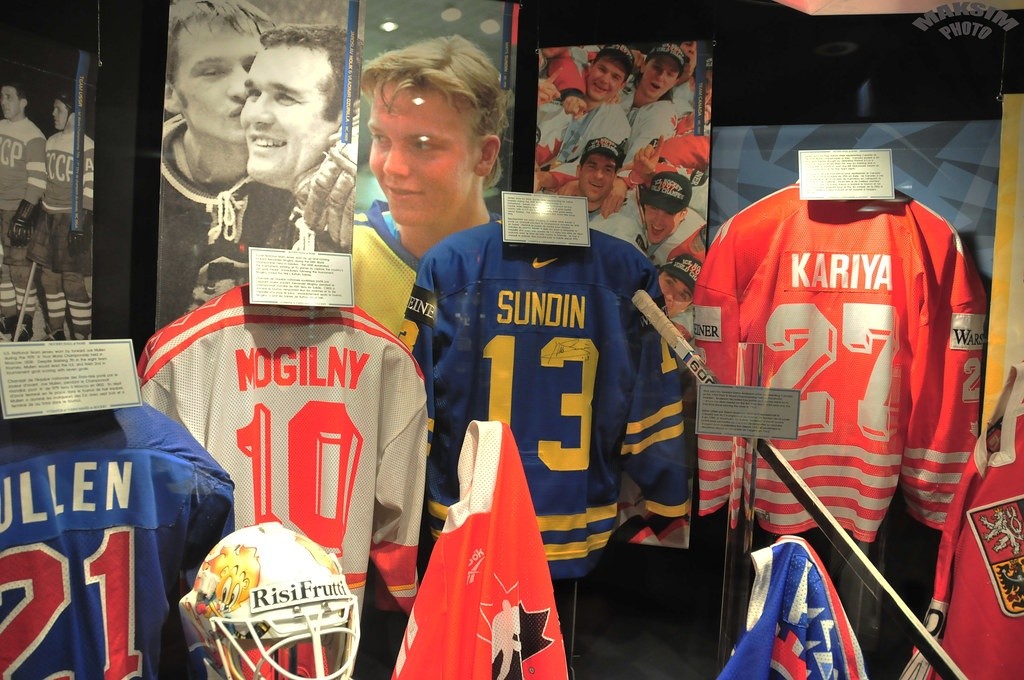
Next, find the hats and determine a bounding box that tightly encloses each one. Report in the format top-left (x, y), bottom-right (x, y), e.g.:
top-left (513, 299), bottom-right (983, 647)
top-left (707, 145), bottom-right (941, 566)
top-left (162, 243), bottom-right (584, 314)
top-left (594, 43), bottom-right (634, 81)
top-left (660, 254), bottom-right (704, 295)
top-left (580, 137), bottom-right (623, 174)
top-left (643, 170), bottom-right (692, 213)
top-left (645, 43), bottom-right (685, 79)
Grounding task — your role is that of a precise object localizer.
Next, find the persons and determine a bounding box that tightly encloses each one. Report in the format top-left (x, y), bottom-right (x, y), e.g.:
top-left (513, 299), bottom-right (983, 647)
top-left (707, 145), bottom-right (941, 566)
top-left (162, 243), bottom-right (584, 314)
top-left (0, 81), bottom-right (95, 342)
top-left (156, 1), bottom-right (360, 329)
top-left (534, 42), bottom-right (716, 337)
top-left (349, 37), bottom-right (510, 336)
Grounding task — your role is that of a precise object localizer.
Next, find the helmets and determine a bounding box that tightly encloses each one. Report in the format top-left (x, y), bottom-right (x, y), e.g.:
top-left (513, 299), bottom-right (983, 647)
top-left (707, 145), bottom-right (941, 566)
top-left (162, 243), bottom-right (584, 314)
top-left (180, 521), bottom-right (362, 680)
top-left (55, 83), bottom-right (76, 112)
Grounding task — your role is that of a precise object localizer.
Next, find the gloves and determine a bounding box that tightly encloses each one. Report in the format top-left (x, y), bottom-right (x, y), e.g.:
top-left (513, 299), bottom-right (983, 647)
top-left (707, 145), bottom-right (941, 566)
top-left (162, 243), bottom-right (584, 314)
top-left (68, 209), bottom-right (94, 249)
top-left (8, 200), bottom-right (38, 246)
